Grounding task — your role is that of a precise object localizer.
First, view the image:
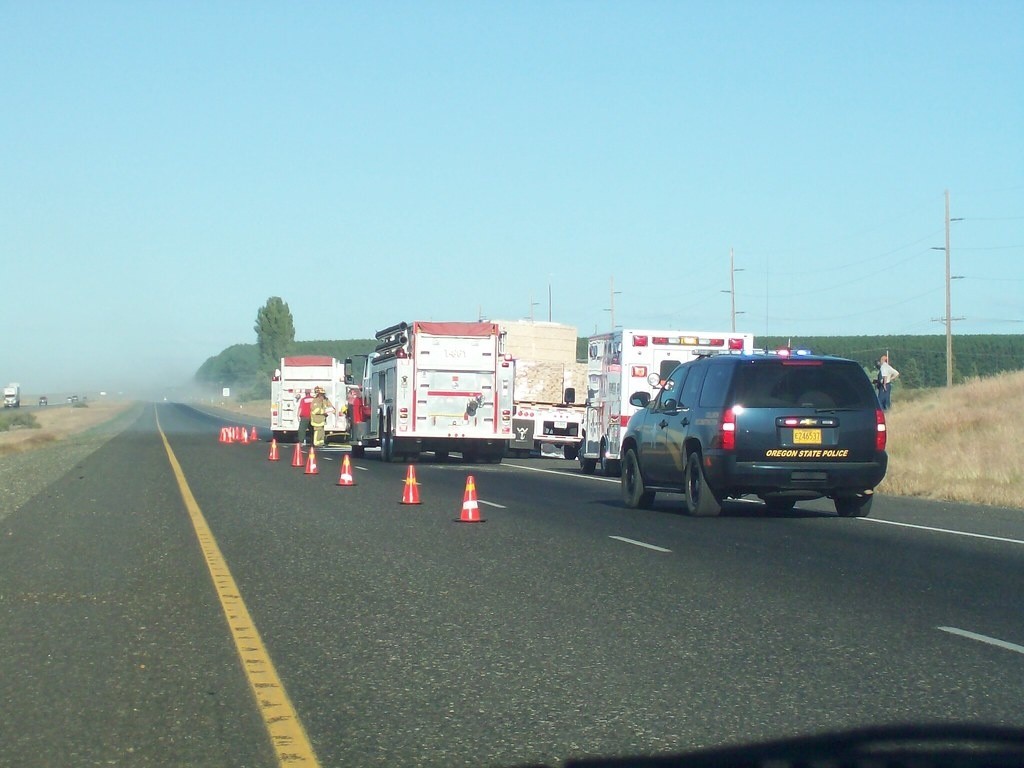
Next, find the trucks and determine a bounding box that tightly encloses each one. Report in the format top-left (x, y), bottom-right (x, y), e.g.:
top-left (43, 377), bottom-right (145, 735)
top-left (4, 383), bottom-right (20, 408)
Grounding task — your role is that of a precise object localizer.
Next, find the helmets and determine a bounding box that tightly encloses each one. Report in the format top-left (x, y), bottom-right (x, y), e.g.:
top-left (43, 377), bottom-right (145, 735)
top-left (316, 389), bottom-right (325, 393)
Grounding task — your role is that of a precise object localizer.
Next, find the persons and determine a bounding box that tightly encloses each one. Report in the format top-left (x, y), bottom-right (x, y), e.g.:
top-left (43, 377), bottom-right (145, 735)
top-left (867, 354), bottom-right (899, 410)
top-left (341, 387), bottom-right (366, 457)
top-left (296, 389), bottom-right (316, 446)
top-left (308, 386), bottom-right (335, 447)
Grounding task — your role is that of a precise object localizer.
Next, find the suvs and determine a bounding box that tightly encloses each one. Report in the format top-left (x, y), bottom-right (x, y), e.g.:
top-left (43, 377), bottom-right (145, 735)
top-left (619, 354), bottom-right (888, 518)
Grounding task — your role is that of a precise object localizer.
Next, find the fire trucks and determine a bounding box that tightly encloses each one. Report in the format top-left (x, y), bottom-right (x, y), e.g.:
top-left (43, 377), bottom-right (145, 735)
top-left (348, 321), bottom-right (814, 478)
top-left (271, 356), bottom-right (349, 442)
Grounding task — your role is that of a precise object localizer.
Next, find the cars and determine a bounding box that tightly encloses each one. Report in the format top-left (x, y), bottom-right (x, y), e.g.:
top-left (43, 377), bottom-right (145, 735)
top-left (39, 397), bottom-right (47, 406)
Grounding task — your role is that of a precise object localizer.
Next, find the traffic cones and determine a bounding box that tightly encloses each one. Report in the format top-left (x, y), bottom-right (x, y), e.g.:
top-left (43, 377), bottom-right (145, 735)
top-left (304, 447), bottom-right (319, 474)
top-left (398, 465), bottom-right (423, 505)
top-left (268, 439), bottom-right (280, 460)
top-left (336, 454), bottom-right (357, 486)
top-left (291, 443), bottom-right (304, 467)
top-left (453, 472), bottom-right (488, 522)
top-left (219, 426), bottom-right (258, 445)
